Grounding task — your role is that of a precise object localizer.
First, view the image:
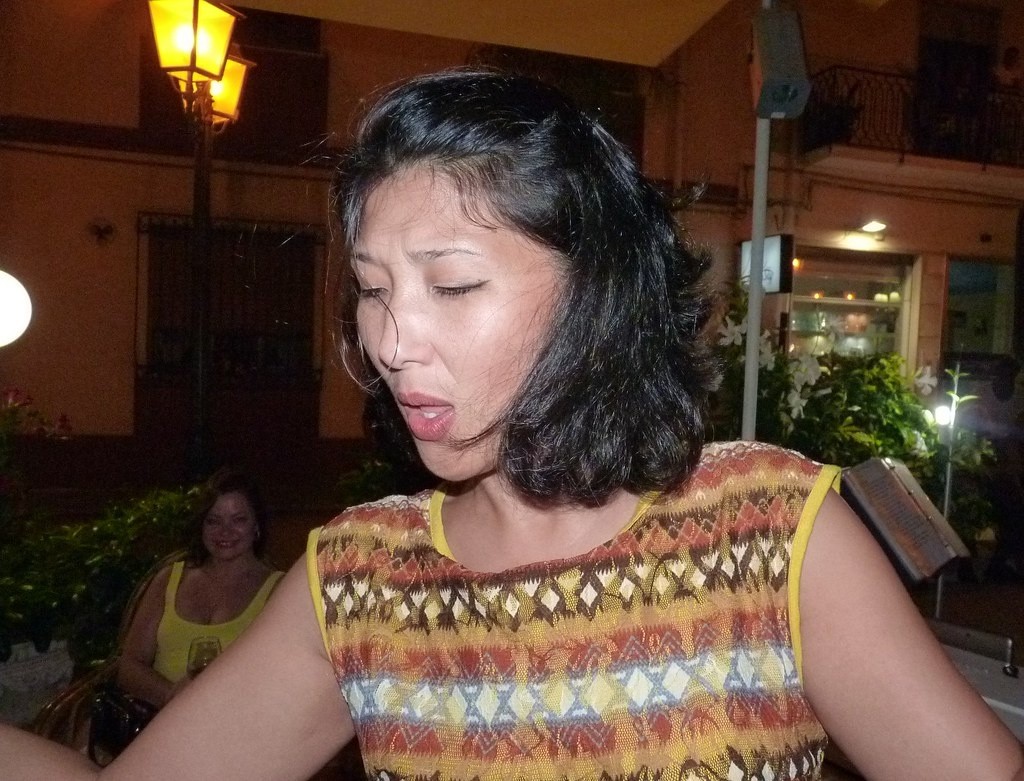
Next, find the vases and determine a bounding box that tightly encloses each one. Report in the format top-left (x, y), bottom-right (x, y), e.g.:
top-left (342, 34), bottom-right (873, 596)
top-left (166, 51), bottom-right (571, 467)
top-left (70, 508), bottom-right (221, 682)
top-left (0, 640), bottom-right (77, 729)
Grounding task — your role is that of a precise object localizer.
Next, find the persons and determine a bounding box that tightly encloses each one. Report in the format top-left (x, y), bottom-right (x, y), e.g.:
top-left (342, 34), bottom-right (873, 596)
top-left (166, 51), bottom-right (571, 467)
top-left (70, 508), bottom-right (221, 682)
top-left (0, 67), bottom-right (1024, 781)
top-left (115, 470), bottom-right (285, 710)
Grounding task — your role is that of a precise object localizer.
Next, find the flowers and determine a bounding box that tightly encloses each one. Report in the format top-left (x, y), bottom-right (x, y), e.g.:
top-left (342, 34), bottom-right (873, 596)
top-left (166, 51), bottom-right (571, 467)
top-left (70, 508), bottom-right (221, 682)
top-left (0, 385), bottom-right (204, 678)
top-left (696, 299), bottom-right (1000, 545)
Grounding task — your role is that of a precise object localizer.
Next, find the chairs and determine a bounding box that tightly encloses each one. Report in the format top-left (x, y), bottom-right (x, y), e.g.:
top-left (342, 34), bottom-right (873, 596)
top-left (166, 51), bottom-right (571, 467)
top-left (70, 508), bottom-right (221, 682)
top-left (31, 548), bottom-right (188, 747)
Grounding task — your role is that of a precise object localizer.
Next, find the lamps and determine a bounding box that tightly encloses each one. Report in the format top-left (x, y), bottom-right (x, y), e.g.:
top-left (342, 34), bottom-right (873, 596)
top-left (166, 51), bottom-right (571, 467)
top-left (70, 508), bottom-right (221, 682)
top-left (863, 216), bottom-right (892, 242)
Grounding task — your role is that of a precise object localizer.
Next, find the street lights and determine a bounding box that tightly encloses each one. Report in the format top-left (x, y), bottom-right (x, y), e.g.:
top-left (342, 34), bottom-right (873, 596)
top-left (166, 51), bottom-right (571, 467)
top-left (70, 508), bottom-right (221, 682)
top-left (935, 350), bottom-right (980, 620)
top-left (149, 0), bottom-right (258, 488)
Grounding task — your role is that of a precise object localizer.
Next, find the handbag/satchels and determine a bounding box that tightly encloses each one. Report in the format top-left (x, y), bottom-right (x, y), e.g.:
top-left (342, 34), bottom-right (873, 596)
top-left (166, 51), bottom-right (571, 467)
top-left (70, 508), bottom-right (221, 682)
top-left (87, 682), bottom-right (159, 768)
